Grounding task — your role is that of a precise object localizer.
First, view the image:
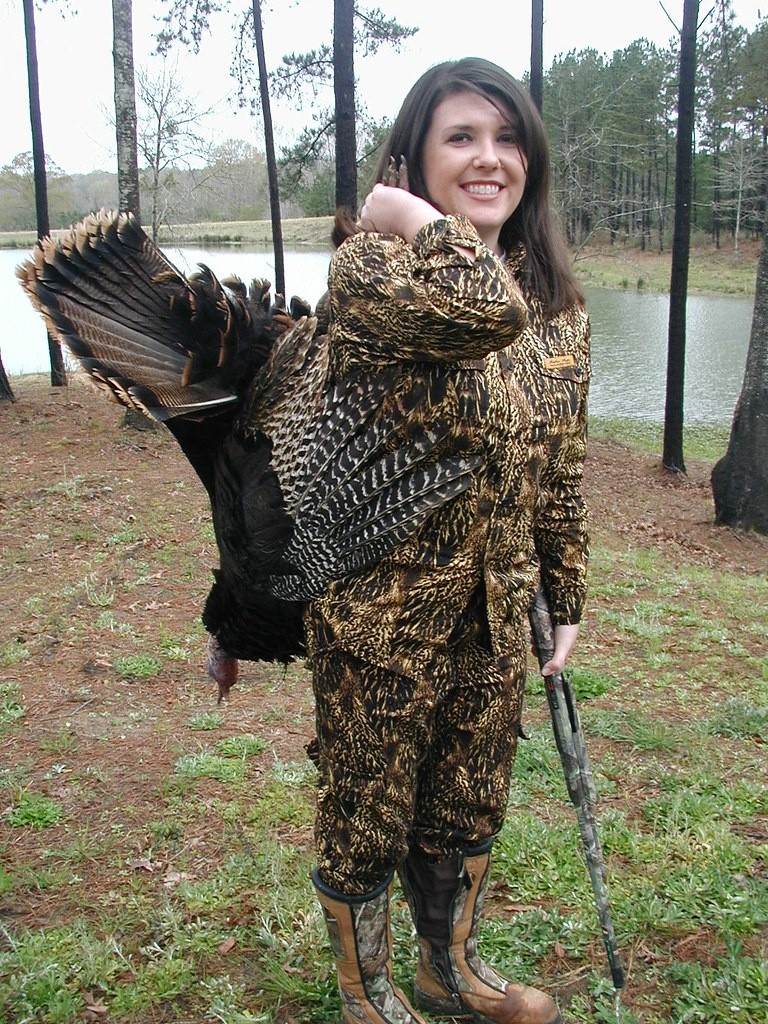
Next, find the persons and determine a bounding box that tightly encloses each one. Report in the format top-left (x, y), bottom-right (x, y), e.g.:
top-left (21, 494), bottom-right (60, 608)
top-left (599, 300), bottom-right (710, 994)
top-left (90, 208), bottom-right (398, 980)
top-left (304, 57), bottom-right (593, 1024)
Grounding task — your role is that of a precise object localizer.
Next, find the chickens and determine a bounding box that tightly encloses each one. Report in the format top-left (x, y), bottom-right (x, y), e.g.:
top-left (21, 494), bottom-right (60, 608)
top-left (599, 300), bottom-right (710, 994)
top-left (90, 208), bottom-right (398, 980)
top-left (14, 212), bottom-right (317, 702)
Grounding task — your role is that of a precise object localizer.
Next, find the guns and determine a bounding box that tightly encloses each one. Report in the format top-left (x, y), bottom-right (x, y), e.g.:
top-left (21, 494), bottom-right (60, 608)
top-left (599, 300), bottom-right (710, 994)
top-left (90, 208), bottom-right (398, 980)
top-left (526, 584), bottom-right (632, 992)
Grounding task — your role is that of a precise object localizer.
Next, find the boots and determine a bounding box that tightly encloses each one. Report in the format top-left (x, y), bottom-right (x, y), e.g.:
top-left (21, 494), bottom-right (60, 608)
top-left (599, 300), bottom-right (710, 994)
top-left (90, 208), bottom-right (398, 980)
top-left (395, 837), bottom-right (560, 1024)
top-left (312, 865), bottom-right (427, 1024)
